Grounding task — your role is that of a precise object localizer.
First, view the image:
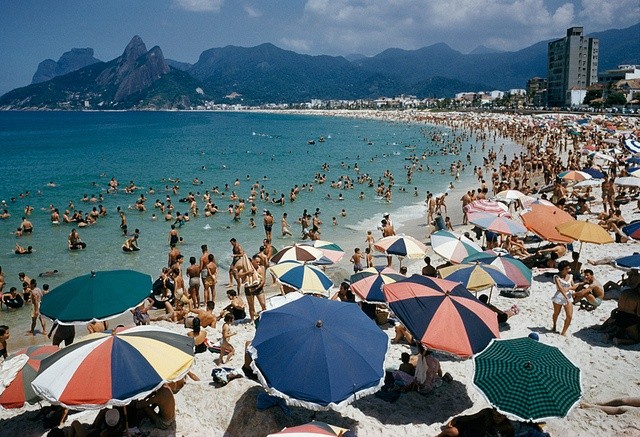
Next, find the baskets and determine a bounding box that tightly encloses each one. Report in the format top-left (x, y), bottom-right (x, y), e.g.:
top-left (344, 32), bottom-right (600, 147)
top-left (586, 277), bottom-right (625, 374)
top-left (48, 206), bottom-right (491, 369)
top-left (376, 311), bottom-right (389, 324)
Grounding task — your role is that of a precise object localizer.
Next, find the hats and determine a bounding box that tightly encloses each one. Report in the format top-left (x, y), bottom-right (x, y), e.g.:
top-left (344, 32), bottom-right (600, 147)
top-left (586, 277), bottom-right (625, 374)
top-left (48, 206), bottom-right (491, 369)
top-left (105, 409), bottom-right (120, 426)
top-left (383, 212), bottom-right (390, 217)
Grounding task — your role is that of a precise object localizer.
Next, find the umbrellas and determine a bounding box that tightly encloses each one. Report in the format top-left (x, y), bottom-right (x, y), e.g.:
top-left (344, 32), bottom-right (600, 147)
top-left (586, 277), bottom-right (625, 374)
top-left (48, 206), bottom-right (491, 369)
top-left (471, 336), bottom-right (584, 423)
top-left (305, 240), bottom-right (345, 266)
top-left (246, 295), bottom-right (391, 412)
top-left (554, 218), bottom-right (614, 277)
top-left (266, 420), bottom-right (357, 437)
top-left (557, 170), bottom-right (592, 181)
top-left (242, 253), bottom-right (262, 319)
top-left (460, 249), bottom-right (533, 305)
top-left (620, 221), bottom-right (640, 243)
top-left (382, 273), bottom-right (500, 361)
top-left (30, 324), bottom-right (195, 437)
top-left (39, 268), bottom-right (153, 331)
top-left (0, 345), bottom-right (60, 409)
top-left (614, 253), bottom-right (640, 270)
top-left (269, 259), bottom-right (334, 295)
top-left (622, 138), bottom-right (640, 155)
top-left (374, 233), bottom-right (429, 268)
top-left (350, 265), bottom-right (406, 314)
top-left (462, 190), bottom-right (555, 235)
top-left (437, 260), bottom-right (517, 298)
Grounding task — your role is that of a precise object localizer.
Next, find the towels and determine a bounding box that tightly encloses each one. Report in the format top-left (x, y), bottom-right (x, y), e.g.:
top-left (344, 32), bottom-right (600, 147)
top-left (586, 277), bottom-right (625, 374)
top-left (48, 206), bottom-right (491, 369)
top-left (414, 353), bottom-right (428, 385)
top-left (235, 253), bottom-right (262, 287)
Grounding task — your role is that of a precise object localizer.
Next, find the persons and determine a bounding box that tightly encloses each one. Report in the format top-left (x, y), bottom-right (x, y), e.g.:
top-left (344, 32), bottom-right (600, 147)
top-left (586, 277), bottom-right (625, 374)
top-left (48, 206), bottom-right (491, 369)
top-left (552, 261), bottom-right (576, 336)
top-left (604, 269), bottom-right (640, 300)
top-left (399, 352), bottom-right (415, 376)
top-left (430, 229), bottom-right (483, 265)
top-left (262, 238), bottom-right (276, 286)
top-left (0, 266), bottom-right (32, 310)
top-left (566, 148), bottom-right (640, 170)
top-left (321, 148), bottom-right (461, 233)
top-left (615, 221), bottom-right (640, 243)
top-left (390, 325), bottom-right (417, 347)
top-left (241, 317), bottom-right (259, 380)
top-left (365, 231), bottom-right (375, 252)
top-left (346, 291), bottom-right (356, 303)
top-left (186, 256), bottom-right (200, 309)
top-left (269, 243), bottom-right (324, 265)
top-left (199, 244), bottom-right (215, 302)
top-left (330, 282), bottom-right (350, 301)
top-left (170, 255), bottom-right (184, 277)
top-left (1, 147), bottom-right (326, 255)
top-left (231, 257), bottom-right (251, 295)
top-left (554, 181), bottom-right (591, 230)
top-left (42, 284), bottom-right (50, 295)
top-left (528, 333), bottom-right (540, 342)
top-left (26, 404), bottom-right (69, 427)
top-left (99, 406), bottom-right (126, 437)
top-left (46, 419), bottom-right (107, 437)
top-left (461, 148), bottom-right (566, 192)
top-left (349, 248), bottom-right (365, 272)
top-left (150, 301), bottom-right (188, 323)
top-left (29, 278), bottom-right (47, 336)
top-left (162, 269), bottom-right (170, 301)
top-left (618, 170), bottom-right (640, 214)
top-left (603, 268), bottom-right (634, 288)
top-left (169, 371), bottom-right (200, 394)
top-left (517, 203), bottom-right (576, 254)
top-left (48, 322), bottom-right (75, 347)
top-left (179, 299), bottom-right (217, 328)
top-left (226, 237), bottom-right (244, 288)
top-left (483, 230), bottom-right (530, 257)
top-left (460, 187), bottom-right (489, 225)
top-left (422, 349), bottom-right (442, 392)
top-left (623, 117), bottom-right (640, 141)
top-left (399, 266), bottom-right (407, 276)
top-left (422, 256), bottom-right (435, 277)
top-left (381, 219), bottom-right (396, 268)
top-left (173, 268), bottom-right (185, 306)
top-left (257, 246), bottom-right (268, 287)
top-left (574, 268), bottom-right (605, 306)
top-left (0, 325), bottom-right (9, 359)
top-left (364, 248), bottom-right (374, 268)
top-left (217, 312), bottom-right (237, 365)
top-left (87, 321), bottom-right (108, 334)
top-left (479, 294), bottom-right (518, 324)
top-left (201, 253), bottom-right (216, 308)
top-left (238, 254), bottom-right (267, 321)
top-left (601, 282), bottom-right (640, 346)
top-left (168, 243), bottom-right (179, 281)
top-left (363, 111), bottom-right (623, 148)
top-left (162, 267), bottom-right (167, 277)
top-left (597, 175), bottom-right (625, 231)
top-left (218, 289), bottom-right (247, 320)
top-left (361, 301), bottom-right (387, 321)
top-left (187, 317), bottom-right (207, 354)
top-left (568, 251), bottom-right (582, 279)
top-left (138, 385), bottom-right (175, 430)
top-left (436, 407), bottom-right (515, 437)
top-left (580, 396), bottom-right (640, 415)
